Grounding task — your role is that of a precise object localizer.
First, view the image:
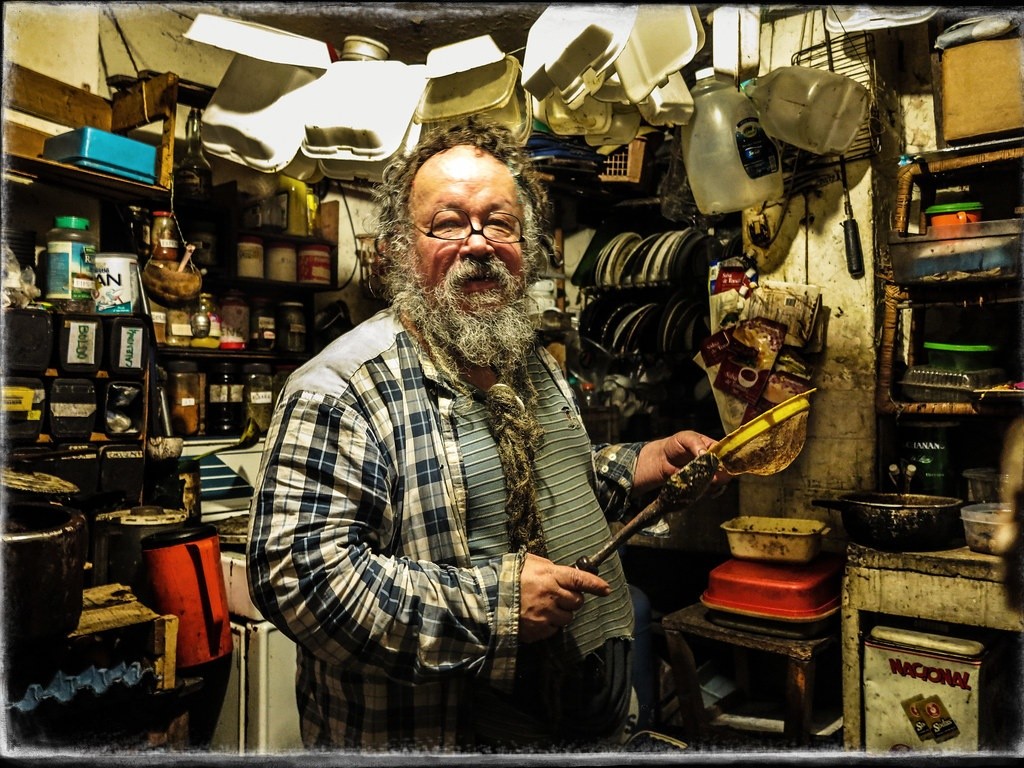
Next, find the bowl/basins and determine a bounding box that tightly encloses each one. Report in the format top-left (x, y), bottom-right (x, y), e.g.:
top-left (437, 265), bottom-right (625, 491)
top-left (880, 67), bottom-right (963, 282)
top-left (958, 501), bottom-right (1020, 556)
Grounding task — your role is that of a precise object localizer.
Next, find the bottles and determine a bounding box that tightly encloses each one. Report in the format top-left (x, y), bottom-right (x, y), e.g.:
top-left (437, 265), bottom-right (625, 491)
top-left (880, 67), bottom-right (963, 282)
top-left (141, 206), bottom-right (337, 438)
top-left (679, 61), bottom-right (871, 214)
top-left (46, 216), bottom-right (101, 316)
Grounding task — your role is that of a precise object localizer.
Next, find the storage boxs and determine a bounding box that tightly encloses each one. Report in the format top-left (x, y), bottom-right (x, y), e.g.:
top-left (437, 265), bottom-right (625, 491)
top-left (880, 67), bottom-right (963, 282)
top-left (943, 36), bottom-right (1023, 141)
top-left (889, 218), bottom-right (1024, 281)
top-left (46, 124), bottom-right (156, 183)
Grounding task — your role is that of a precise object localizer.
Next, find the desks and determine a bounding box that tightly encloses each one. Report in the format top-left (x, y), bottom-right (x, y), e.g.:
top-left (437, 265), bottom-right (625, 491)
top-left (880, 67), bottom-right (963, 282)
top-left (663, 605), bottom-right (835, 754)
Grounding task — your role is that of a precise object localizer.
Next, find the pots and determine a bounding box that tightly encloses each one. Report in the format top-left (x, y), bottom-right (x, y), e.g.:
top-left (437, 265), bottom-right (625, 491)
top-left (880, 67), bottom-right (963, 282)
top-left (811, 490), bottom-right (968, 552)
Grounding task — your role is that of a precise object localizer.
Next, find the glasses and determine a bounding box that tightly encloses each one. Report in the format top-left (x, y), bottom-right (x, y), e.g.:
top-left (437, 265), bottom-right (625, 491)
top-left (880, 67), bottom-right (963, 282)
top-left (411, 209), bottom-right (527, 244)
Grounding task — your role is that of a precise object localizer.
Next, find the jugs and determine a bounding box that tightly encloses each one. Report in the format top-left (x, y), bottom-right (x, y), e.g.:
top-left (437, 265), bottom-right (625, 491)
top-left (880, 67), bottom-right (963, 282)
top-left (136, 526), bottom-right (233, 667)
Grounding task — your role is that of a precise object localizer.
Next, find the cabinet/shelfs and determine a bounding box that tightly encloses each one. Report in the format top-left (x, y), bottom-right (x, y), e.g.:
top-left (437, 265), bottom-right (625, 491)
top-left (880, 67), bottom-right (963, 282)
top-left (877, 144), bottom-right (1024, 412)
top-left (194, 546), bottom-right (304, 753)
top-left (3, 59), bottom-right (340, 753)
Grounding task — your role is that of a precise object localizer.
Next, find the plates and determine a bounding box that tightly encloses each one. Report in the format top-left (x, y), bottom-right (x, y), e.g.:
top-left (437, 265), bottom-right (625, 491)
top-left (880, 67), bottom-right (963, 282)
top-left (568, 225), bottom-right (712, 360)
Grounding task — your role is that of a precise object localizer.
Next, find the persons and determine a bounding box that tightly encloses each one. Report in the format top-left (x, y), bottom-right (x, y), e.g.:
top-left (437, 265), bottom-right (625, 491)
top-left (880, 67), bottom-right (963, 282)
top-left (243, 112), bottom-right (721, 754)
